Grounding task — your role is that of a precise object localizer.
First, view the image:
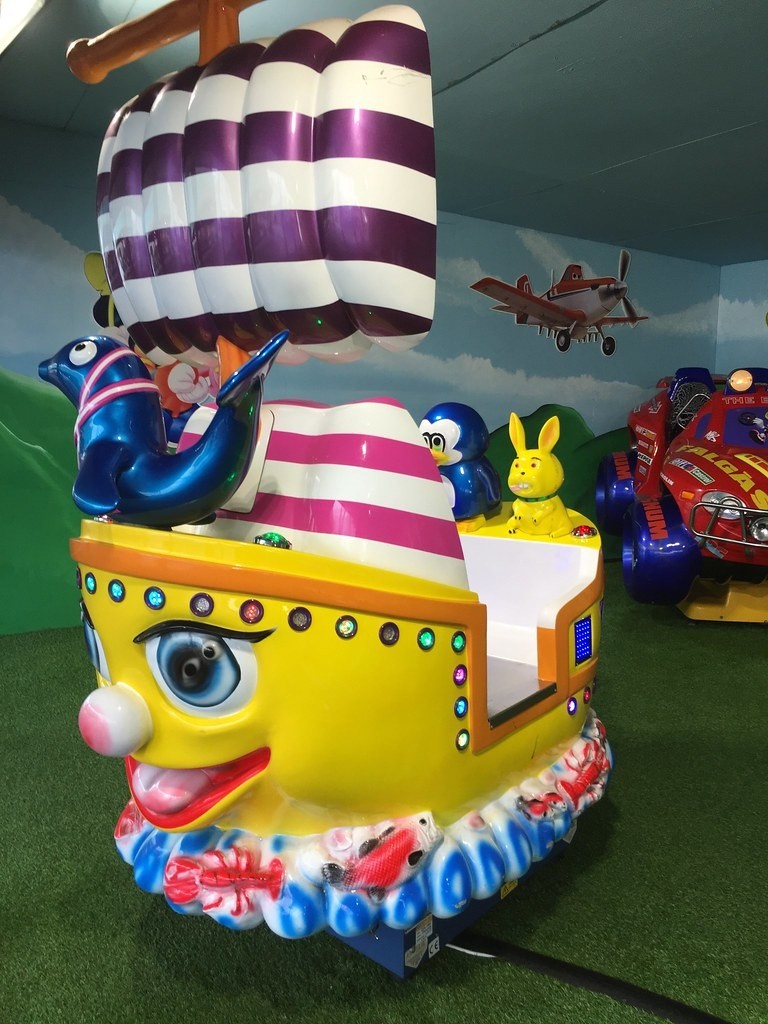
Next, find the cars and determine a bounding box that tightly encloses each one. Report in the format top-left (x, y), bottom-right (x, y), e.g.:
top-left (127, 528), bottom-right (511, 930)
top-left (595, 365), bottom-right (768, 605)
